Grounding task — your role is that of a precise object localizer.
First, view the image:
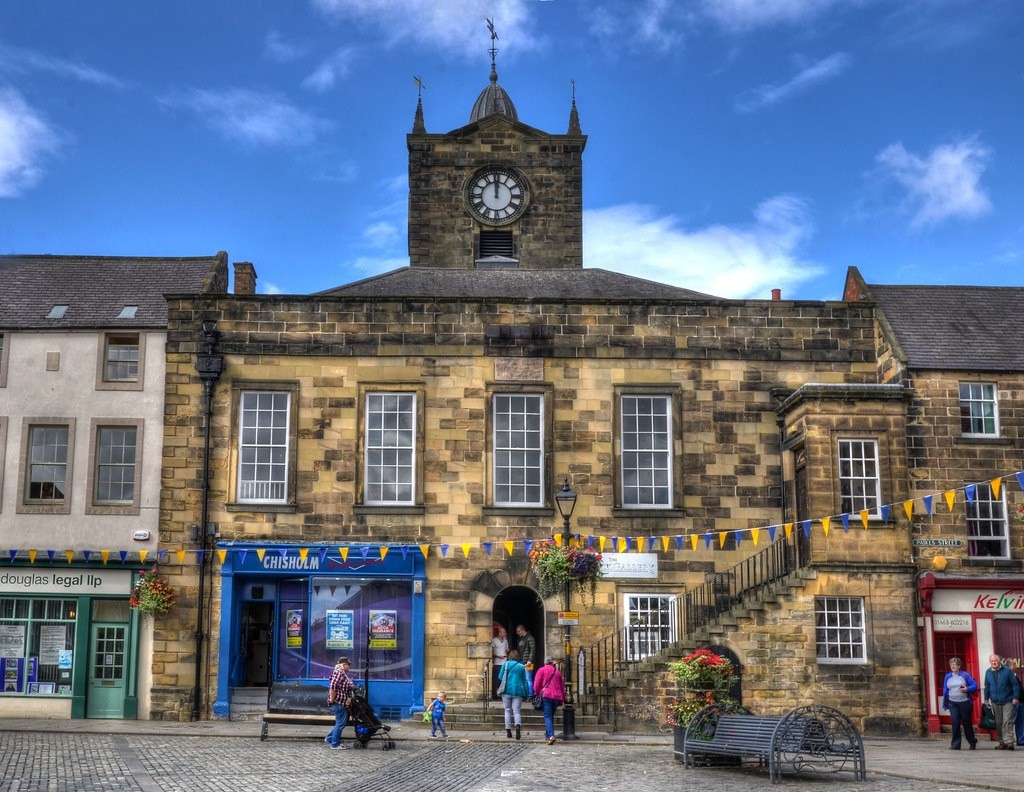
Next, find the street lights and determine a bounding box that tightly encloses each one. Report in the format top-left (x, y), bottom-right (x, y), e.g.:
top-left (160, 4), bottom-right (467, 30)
top-left (555, 475), bottom-right (579, 739)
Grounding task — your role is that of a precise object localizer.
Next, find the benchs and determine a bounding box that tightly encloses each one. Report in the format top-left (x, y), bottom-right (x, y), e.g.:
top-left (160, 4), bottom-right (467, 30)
top-left (259, 682), bottom-right (362, 741)
top-left (682, 703), bottom-right (867, 785)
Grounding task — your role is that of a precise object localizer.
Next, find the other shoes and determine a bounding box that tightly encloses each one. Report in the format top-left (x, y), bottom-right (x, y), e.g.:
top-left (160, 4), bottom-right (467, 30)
top-left (548, 736), bottom-right (556, 744)
top-left (995, 743), bottom-right (1006, 750)
top-left (526, 695), bottom-right (536, 703)
top-left (331, 743), bottom-right (347, 750)
top-left (430, 734), bottom-right (438, 738)
top-left (324, 738), bottom-right (331, 746)
top-left (948, 746), bottom-right (961, 750)
top-left (506, 729), bottom-right (512, 738)
top-left (1007, 743), bottom-right (1015, 750)
top-left (515, 724), bottom-right (521, 740)
top-left (969, 738), bottom-right (978, 750)
top-left (443, 735), bottom-right (451, 739)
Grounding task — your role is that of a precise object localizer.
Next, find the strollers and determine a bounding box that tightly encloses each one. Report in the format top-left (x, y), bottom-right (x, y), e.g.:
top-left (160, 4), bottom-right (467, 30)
top-left (346, 684), bottom-right (396, 751)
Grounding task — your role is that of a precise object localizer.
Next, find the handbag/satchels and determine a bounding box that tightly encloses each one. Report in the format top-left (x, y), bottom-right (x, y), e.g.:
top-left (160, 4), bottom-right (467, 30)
top-left (978, 704), bottom-right (997, 730)
top-left (497, 662), bottom-right (508, 697)
top-left (423, 711), bottom-right (432, 724)
top-left (532, 694), bottom-right (543, 710)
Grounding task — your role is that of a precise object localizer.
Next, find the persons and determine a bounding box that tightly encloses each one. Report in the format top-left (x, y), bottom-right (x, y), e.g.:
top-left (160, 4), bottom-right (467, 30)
top-left (426, 692), bottom-right (450, 738)
top-left (324, 658), bottom-right (355, 750)
top-left (517, 626), bottom-right (535, 701)
top-left (492, 627), bottom-right (509, 701)
top-left (534, 657), bottom-right (564, 744)
top-left (943, 658), bottom-right (978, 750)
top-left (499, 651), bottom-right (526, 740)
top-left (984, 656), bottom-right (1018, 749)
top-left (1008, 662), bottom-right (1024, 746)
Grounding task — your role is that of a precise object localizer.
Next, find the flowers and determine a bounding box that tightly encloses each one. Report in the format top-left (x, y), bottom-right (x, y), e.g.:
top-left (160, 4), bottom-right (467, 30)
top-left (534, 542), bottom-right (607, 611)
top-left (665, 688), bottom-right (749, 741)
top-left (663, 649), bottom-right (740, 695)
top-left (128, 568), bottom-right (176, 622)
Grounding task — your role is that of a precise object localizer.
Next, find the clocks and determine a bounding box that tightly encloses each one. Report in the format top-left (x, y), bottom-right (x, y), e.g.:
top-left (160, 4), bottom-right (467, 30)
top-left (465, 167), bottom-right (527, 222)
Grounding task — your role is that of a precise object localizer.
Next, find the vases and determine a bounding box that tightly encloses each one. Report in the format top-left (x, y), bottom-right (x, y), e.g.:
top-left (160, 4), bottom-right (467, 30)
top-left (540, 554), bottom-right (599, 583)
top-left (672, 726), bottom-right (743, 767)
